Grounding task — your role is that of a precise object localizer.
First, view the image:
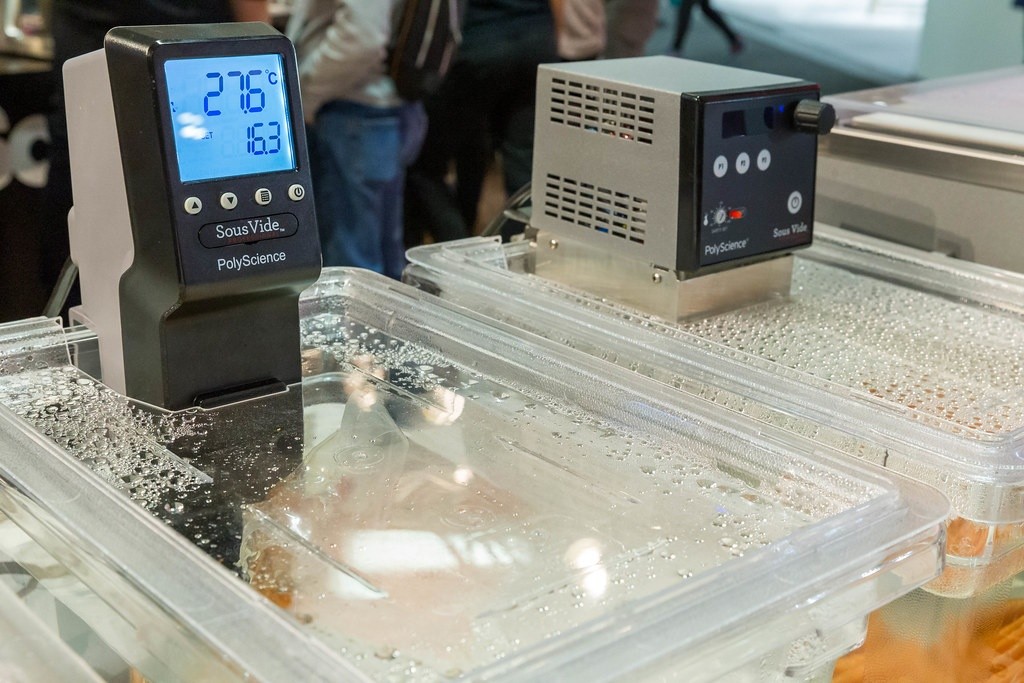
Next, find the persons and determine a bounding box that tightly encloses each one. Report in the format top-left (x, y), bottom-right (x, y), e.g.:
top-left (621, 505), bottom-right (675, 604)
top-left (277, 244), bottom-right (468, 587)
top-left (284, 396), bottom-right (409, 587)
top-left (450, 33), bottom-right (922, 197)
top-left (45, 0), bottom-right (659, 322)
top-left (672, 0), bottom-right (744, 55)
